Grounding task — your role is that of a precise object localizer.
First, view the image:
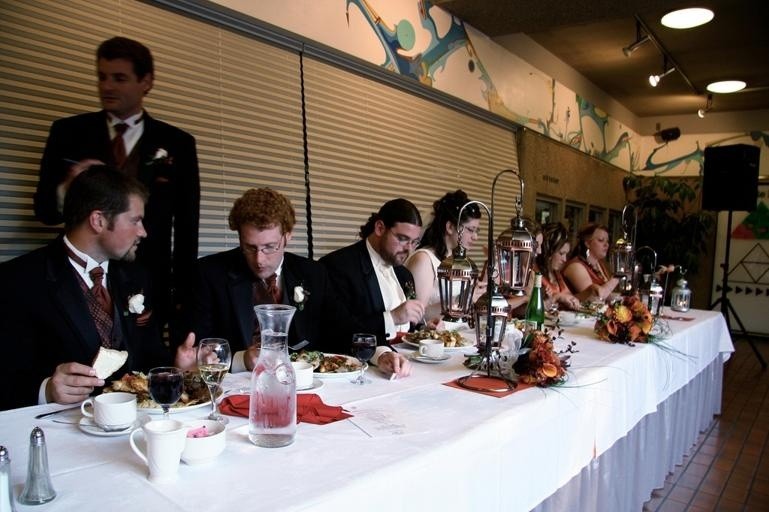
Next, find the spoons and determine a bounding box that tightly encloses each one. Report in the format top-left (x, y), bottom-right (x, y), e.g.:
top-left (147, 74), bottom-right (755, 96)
top-left (51, 419), bottom-right (132, 430)
top-left (409, 356), bottom-right (450, 360)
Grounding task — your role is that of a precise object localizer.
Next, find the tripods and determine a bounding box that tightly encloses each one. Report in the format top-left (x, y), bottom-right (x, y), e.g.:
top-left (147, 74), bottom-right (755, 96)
top-left (707, 213), bottom-right (764, 371)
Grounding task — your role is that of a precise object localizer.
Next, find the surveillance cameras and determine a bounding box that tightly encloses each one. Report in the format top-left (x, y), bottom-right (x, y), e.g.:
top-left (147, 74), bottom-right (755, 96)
top-left (652, 126), bottom-right (681, 144)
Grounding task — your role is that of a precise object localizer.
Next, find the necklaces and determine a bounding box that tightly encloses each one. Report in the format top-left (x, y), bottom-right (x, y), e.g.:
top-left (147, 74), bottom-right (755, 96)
top-left (587, 261), bottom-right (605, 280)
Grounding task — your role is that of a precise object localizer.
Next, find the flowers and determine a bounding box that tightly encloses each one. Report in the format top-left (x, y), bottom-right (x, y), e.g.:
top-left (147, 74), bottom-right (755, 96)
top-left (594, 294), bottom-right (670, 350)
top-left (462, 324), bottom-right (579, 389)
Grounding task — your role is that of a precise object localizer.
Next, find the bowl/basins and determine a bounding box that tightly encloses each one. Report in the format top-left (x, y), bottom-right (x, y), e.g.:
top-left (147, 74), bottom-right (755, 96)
top-left (182, 422), bottom-right (226, 464)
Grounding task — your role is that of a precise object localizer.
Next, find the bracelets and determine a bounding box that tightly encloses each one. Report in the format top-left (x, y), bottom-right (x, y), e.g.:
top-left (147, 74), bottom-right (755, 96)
top-left (606, 280), bottom-right (613, 292)
top-left (543, 297), bottom-right (558, 315)
top-left (475, 279), bottom-right (486, 287)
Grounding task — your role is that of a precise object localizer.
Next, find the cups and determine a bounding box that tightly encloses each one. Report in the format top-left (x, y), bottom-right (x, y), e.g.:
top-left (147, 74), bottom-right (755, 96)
top-left (130, 419), bottom-right (186, 482)
top-left (81, 392), bottom-right (137, 428)
top-left (292, 361), bottom-right (313, 385)
top-left (420, 339), bottom-right (445, 358)
top-left (149, 369), bottom-right (185, 418)
top-left (353, 333), bottom-right (376, 385)
top-left (198, 339), bottom-right (231, 420)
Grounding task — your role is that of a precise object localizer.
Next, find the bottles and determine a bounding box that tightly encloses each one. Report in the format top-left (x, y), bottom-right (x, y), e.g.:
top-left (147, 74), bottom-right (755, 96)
top-left (524, 271), bottom-right (544, 347)
top-left (0, 445), bottom-right (17, 512)
top-left (17, 426), bottom-right (57, 506)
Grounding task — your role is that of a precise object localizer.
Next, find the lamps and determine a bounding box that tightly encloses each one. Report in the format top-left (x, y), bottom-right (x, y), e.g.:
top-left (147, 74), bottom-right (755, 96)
top-left (648, 67), bottom-right (677, 87)
top-left (697, 94), bottom-right (713, 119)
top-left (660, 7), bottom-right (716, 30)
top-left (622, 36), bottom-right (650, 59)
top-left (705, 80), bottom-right (746, 94)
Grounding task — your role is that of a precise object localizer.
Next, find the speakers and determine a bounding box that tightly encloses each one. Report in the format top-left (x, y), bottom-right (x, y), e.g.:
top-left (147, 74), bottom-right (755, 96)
top-left (701, 144), bottom-right (760, 211)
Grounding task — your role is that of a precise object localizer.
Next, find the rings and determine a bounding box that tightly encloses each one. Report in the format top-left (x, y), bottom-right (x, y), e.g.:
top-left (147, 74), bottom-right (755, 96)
top-left (571, 297), bottom-right (575, 301)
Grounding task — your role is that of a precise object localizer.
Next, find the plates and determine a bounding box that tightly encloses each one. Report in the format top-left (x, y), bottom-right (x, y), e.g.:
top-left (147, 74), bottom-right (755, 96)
top-left (296, 379), bottom-right (325, 391)
top-left (410, 351), bottom-right (452, 363)
top-left (103, 382), bottom-right (224, 412)
top-left (78, 414), bottom-right (152, 435)
top-left (289, 353), bottom-right (368, 380)
top-left (401, 333), bottom-right (474, 348)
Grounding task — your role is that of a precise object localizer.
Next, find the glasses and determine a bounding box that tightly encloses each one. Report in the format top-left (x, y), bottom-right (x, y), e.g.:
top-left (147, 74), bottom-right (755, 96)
top-left (387, 226), bottom-right (422, 248)
top-left (242, 234), bottom-right (285, 255)
top-left (458, 222), bottom-right (481, 235)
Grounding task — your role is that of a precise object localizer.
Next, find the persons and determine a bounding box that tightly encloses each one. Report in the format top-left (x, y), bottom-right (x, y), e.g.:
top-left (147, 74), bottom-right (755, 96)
top-left (33, 37), bottom-right (200, 328)
top-left (160, 189), bottom-right (413, 379)
top-left (531, 222), bottom-right (584, 310)
top-left (403, 189), bottom-right (488, 324)
top-left (560, 223), bottom-right (640, 310)
top-left (319, 198), bottom-right (445, 344)
top-left (0, 167), bottom-right (169, 411)
top-left (480, 217), bottom-right (569, 311)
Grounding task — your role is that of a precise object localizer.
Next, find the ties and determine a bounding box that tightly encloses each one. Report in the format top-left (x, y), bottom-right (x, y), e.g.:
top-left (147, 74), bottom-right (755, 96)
top-left (106, 111), bottom-right (144, 166)
top-left (65, 243), bottom-right (113, 315)
top-left (266, 272), bottom-right (281, 306)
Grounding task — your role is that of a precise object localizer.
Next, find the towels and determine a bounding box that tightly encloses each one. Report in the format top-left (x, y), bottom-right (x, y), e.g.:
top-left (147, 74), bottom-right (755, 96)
top-left (219, 392), bottom-right (354, 425)
top-left (389, 332), bottom-right (410, 344)
